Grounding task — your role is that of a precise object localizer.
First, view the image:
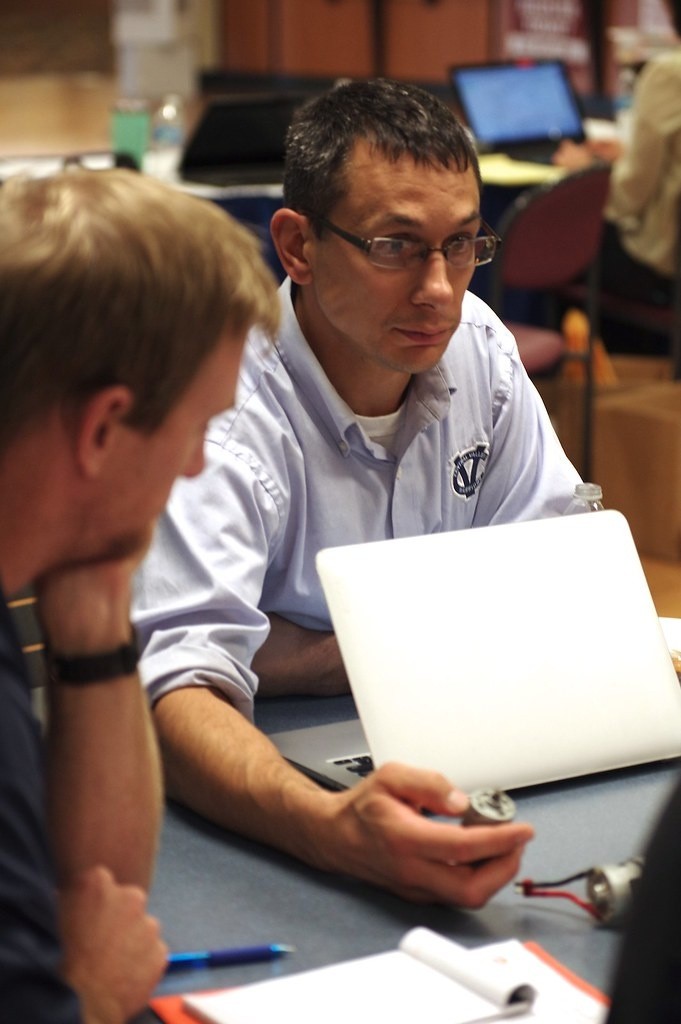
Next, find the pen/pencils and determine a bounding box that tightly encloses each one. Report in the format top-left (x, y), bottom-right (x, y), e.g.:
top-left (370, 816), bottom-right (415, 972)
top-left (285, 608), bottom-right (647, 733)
top-left (169, 944), bottom-right (294, 967)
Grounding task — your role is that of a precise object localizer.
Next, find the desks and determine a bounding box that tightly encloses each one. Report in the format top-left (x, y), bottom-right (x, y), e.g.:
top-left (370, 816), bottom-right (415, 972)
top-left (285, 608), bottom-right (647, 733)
top-left (0, 146), bottom-right (564, 331)
top-left (148, 616), bottom-right (681, 1024)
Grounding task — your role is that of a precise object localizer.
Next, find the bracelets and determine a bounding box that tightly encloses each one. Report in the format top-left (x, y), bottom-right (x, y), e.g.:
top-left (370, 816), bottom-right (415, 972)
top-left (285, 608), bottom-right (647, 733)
top-left (39, 624), bottom-right (143, 685)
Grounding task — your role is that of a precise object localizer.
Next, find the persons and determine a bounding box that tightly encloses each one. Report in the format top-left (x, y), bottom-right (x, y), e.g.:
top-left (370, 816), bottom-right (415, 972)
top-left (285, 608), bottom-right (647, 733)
top-left (553, 0), bottom-right (681, 285)
top-left (131, 82), bottom-right (612, 915)
top-left (0, 164), bottom-right (291, 1024)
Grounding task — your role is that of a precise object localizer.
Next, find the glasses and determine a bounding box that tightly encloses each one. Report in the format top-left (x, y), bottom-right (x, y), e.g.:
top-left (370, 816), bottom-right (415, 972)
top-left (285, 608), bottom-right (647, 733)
top-left (298, 210), bottom-right (503, 270)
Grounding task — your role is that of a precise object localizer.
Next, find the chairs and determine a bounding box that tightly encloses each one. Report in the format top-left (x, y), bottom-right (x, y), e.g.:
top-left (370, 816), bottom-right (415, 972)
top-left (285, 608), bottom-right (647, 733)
top-left (492, 162), bottom-right (612, 485)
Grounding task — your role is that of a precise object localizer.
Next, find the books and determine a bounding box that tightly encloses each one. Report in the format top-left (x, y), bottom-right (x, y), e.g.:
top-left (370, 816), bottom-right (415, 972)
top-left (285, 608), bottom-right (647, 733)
top-left (157, 921), bottom-right (612, 1024)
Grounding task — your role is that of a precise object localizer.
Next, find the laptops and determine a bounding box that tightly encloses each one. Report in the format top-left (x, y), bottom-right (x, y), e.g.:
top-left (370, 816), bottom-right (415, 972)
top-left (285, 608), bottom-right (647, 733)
top-left (177, 88), bottom-right (314, 187)
top-left (447, 57), bottom-right (617, 173)
top-left (264, 509), bottom-right (681, 800)
